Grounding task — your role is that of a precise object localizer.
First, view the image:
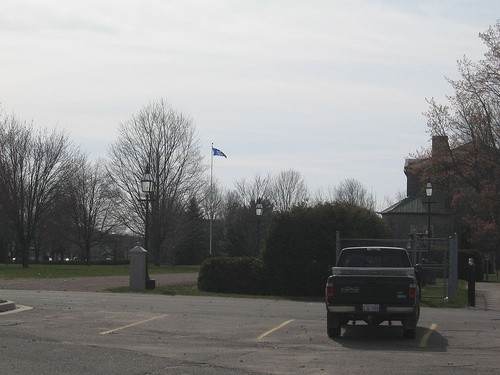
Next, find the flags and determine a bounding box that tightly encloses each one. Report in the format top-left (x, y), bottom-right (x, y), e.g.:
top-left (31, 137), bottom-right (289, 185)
top-left (212, 148), bottom-right (227, 158)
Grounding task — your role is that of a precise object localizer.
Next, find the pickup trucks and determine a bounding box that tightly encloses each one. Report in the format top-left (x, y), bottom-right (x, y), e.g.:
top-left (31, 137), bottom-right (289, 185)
top-left (325, 245), bottom-right (421, 339)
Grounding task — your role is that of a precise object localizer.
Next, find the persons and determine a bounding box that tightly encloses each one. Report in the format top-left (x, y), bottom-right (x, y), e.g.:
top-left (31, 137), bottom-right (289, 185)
top-left (467, 258), bottom-right (476, 307)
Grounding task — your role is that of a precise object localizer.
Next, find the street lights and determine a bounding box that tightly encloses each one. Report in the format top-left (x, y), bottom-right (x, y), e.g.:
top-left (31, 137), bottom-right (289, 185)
top-left (141, 165), bottom-right (155, 280)
top-left (255, 197), bottom-right (265, 255)
top-left (426, 178), bottom-right (434, 258)
